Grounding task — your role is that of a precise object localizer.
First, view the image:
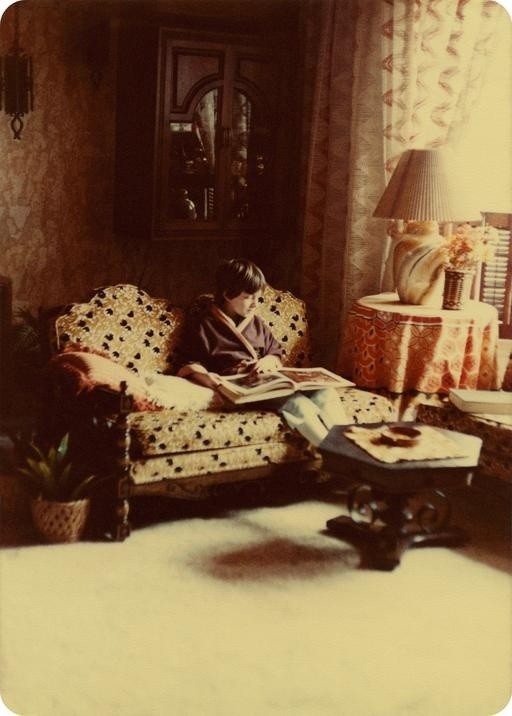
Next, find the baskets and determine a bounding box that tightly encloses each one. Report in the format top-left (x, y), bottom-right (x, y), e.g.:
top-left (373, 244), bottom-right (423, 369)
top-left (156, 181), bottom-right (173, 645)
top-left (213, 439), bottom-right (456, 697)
top-left (31, 497), bottom-right (91, 543)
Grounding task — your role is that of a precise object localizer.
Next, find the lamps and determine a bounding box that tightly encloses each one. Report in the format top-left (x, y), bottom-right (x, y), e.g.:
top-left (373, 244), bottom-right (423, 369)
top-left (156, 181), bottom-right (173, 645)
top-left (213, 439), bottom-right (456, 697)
top-left (372, 149), bottom-right (483, 305)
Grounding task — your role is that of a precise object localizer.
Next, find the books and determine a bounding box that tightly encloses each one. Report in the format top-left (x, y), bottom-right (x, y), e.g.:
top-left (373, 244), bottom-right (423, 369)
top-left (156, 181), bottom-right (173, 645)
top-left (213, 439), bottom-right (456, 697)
top-left (214, 367), bottom-right (358, 406)
top-left (448, 387), bottom-right (512, 415)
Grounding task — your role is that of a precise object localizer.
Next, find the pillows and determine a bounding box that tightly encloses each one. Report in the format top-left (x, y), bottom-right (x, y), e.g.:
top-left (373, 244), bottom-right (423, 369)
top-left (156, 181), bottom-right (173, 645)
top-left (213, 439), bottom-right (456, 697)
top-left (46, 351), bottom-right (225, 412)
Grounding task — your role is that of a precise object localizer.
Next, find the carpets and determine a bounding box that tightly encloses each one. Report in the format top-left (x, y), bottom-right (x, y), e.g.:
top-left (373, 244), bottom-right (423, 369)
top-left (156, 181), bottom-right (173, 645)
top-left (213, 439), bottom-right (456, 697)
top-left (0, 500), bottom-right (512, 715)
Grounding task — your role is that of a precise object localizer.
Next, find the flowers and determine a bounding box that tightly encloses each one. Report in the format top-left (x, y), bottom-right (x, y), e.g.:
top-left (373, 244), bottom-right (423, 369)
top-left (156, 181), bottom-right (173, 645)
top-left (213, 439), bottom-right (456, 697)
top-left (438, 222), bottom-right (500, 272)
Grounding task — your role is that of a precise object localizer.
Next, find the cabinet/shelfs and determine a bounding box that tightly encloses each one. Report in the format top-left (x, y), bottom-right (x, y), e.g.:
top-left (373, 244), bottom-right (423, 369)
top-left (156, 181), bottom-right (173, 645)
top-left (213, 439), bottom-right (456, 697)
top-left (113, 13), bottom-right (295, 243)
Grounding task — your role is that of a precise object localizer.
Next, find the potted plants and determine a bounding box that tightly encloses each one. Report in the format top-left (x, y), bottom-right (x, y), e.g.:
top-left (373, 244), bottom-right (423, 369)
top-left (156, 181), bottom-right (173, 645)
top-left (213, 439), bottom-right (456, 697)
top-left (13, 430), bottom-right (96, 543)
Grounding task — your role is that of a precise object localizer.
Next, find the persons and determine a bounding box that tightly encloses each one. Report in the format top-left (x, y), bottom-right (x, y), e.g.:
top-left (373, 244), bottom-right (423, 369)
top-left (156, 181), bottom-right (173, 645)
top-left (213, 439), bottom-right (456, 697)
top-left (170, 254), bottom-right (375, 514)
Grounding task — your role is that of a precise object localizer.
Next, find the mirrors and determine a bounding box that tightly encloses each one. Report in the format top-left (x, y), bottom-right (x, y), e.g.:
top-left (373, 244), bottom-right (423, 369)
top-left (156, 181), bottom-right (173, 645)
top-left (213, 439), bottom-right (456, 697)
top-left (166, 74), bottom-right (283, 234)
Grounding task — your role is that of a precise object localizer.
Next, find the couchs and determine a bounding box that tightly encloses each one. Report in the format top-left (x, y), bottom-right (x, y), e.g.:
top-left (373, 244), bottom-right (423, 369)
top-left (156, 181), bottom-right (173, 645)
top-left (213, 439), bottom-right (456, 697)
top-left (46, 282), bottom-right (396, 541)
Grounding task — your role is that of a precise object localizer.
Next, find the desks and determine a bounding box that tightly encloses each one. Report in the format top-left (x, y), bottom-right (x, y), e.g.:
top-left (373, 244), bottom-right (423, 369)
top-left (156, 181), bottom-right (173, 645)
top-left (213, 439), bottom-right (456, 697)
top-left (354, 290), bottom-right (498, 422)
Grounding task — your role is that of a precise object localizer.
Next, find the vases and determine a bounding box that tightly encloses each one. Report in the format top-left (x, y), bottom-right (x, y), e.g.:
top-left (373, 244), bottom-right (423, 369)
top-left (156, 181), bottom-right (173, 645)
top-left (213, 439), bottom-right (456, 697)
top-left (442, 270), bottom-right (465, 312)
